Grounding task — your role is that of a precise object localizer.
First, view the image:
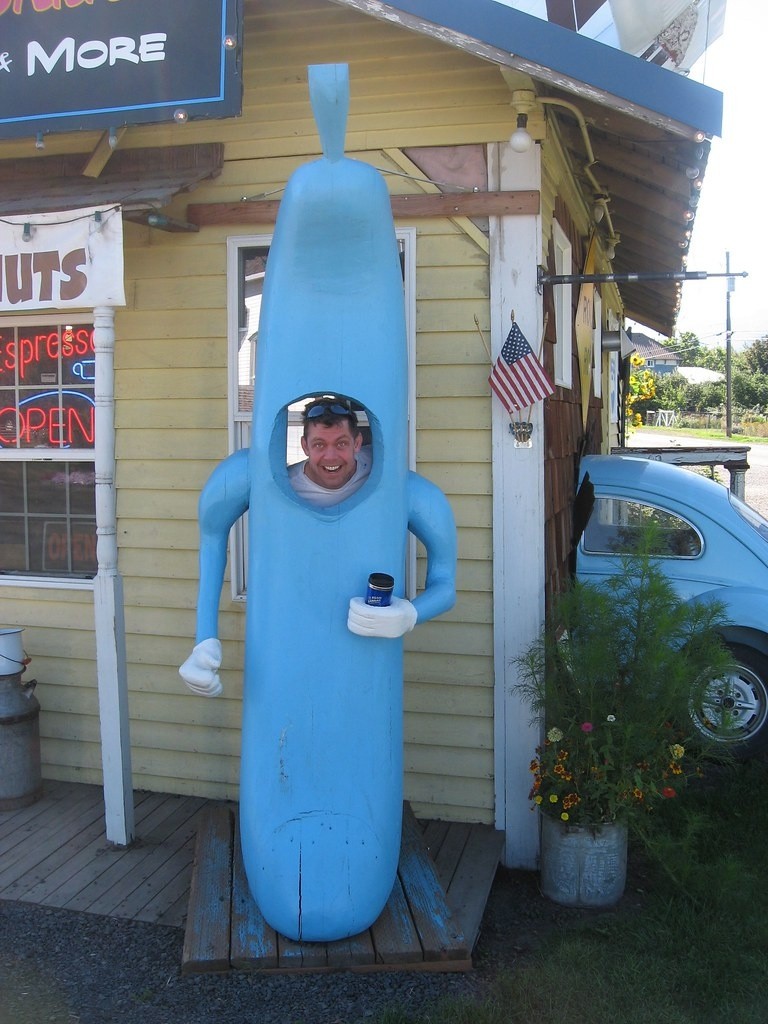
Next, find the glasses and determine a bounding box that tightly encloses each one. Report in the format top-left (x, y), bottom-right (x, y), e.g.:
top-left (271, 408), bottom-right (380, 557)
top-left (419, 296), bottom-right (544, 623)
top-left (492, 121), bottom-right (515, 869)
top-left (304, 405), bottom-right (354, 419)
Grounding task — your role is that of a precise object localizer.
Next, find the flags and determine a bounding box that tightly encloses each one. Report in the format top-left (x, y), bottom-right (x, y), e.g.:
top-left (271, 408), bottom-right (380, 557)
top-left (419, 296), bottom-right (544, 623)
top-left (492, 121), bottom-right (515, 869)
top-left (486, 321), bottom-right (559, 416)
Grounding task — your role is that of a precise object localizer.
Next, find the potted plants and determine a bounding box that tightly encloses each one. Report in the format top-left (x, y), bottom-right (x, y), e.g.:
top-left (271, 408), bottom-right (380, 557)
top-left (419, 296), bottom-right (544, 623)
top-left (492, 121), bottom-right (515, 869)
top-left (510, 510), bottom-right (755, 917)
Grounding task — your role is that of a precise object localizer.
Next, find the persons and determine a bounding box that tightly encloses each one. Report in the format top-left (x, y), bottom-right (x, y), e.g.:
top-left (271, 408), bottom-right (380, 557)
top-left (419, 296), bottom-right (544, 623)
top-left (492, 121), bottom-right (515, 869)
top-left (287, 396), bottom-right (374, 508)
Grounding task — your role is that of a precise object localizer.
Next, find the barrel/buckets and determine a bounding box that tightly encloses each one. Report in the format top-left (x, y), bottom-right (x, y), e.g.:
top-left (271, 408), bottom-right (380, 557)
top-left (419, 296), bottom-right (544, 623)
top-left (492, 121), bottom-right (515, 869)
top-left (0, 628), bottom-right (31, 675)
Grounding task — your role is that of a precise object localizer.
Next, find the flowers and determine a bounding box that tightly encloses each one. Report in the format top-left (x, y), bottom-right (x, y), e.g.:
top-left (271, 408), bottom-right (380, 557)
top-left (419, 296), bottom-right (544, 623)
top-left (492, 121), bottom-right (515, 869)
top-left (526, 714), bottom-right (703, 822)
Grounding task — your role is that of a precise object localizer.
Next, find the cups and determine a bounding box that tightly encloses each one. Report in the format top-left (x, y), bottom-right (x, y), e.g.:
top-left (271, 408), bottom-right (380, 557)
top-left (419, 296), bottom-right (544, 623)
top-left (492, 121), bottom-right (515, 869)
top-left (365, 572), bottom-right (395, 606)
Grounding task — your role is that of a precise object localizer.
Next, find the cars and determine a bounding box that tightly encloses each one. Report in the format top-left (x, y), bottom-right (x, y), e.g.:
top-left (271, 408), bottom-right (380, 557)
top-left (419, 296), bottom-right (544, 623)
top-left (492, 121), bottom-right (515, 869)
top-left (575, 455), bottom-right (768, 761)
